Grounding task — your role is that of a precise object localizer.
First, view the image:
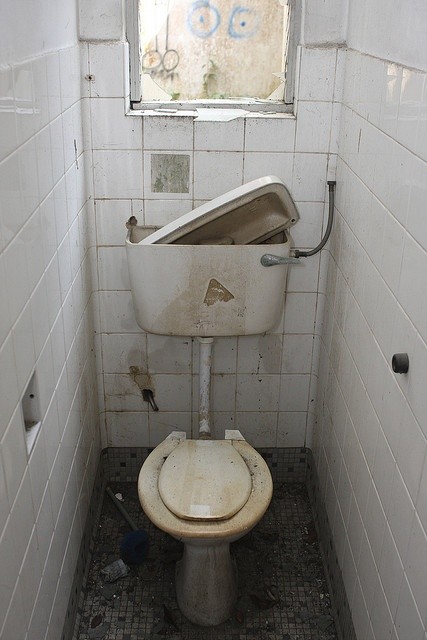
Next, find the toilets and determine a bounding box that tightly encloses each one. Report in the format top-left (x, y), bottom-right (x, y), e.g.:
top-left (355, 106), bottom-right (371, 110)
top-left (121, 174), bottom-right (301, 626)
